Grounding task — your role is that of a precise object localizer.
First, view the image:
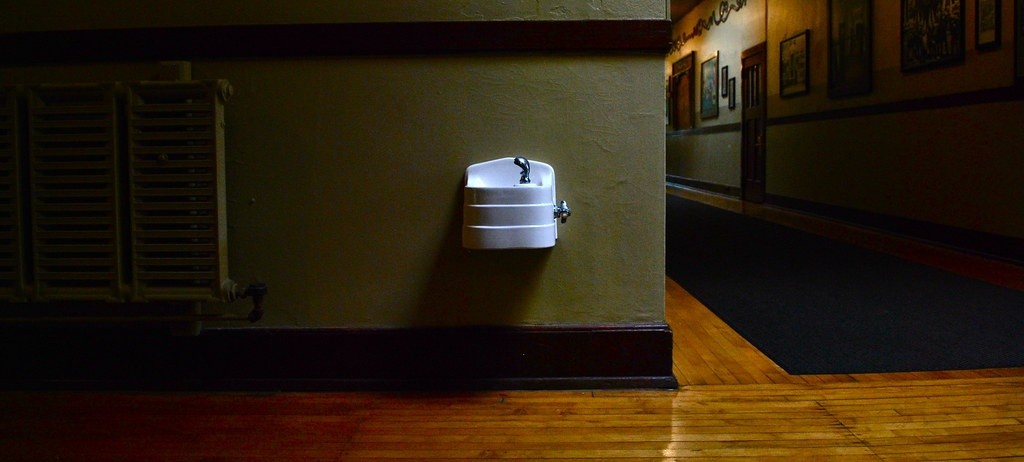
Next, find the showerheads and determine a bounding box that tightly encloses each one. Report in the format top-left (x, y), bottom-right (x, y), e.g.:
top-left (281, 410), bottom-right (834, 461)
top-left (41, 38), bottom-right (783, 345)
top-left (513, 156), bottom-right (531, 184)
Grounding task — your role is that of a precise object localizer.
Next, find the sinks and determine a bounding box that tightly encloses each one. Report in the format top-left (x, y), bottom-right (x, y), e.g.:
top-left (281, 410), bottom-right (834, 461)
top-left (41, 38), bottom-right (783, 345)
top-left (464, 154), bottom-right (558, 250)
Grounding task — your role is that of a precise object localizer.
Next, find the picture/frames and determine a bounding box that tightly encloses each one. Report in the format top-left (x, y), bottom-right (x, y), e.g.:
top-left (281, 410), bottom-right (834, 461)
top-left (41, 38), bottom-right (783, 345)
top-left (700, 51), bottom-right (719, 120)
top-left (976, 0), bottom-right (1002, 50)
top-left (779, 30), bottom-right (809, 99)
top-left (722, 66), bottom-right (727, 96)
top-left (899, 0), bottom-right (966, 73)
top-left (729, 77), bottom-right (735, 109)
top-left (828, 0), bottom-right (873, 95)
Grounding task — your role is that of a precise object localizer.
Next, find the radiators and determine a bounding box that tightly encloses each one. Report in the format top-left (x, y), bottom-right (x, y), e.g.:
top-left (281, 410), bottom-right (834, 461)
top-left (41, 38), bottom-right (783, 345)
top-left (0, 76), bottom-right (239, 307)
